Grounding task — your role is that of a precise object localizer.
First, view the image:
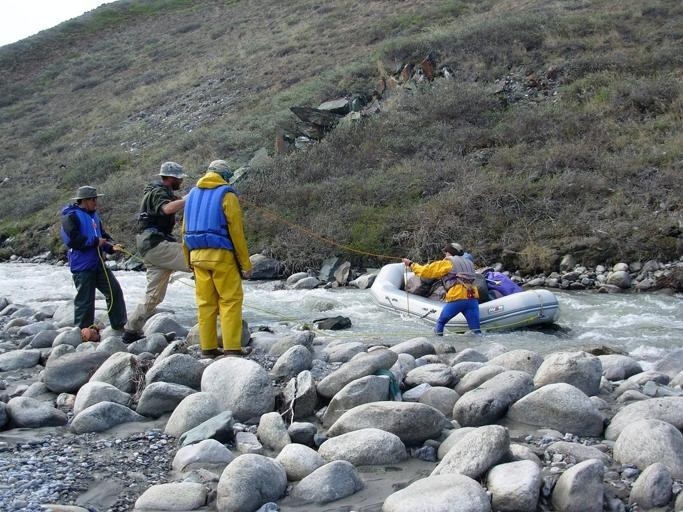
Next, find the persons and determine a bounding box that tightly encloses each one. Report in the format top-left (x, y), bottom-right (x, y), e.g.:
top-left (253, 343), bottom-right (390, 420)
top-left (402, 243), bottom-right (480, 337)
top-left (122, 162), bottom-right (193, 343)
top-left (61, 186), bottom-right (136, 338)
top-left (182, 160), bottom-right (253, 357)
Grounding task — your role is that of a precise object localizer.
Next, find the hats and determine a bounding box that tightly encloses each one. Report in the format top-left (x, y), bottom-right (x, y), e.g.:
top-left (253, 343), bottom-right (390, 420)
top-left (441, 243), bottom-right (464, 257)
top-left (157, 162), bottom-right (189, 179)
top-left (70, 185), bottom-right (105, 200)
top-left (205, 160), bottom-right (234, 179)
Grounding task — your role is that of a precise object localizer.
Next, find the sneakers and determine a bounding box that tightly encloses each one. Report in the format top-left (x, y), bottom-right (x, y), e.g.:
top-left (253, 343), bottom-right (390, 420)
top-left (122, 333), bottom-right (147, 345)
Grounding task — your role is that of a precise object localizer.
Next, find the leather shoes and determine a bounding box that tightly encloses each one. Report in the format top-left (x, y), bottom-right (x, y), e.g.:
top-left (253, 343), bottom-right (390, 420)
top-left (201, 346), bottom-right (253, 359)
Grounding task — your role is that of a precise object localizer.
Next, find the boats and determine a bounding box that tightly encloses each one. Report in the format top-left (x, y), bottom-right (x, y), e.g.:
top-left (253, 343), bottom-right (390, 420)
top-left (369, 260), bottom-right (560, 333)
